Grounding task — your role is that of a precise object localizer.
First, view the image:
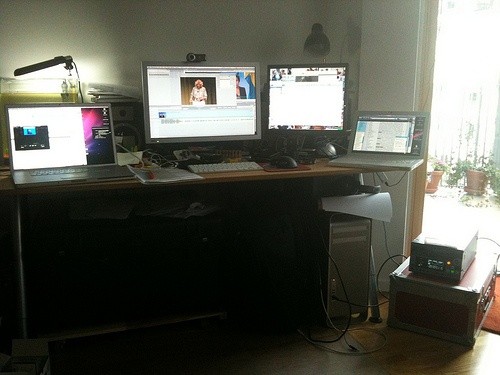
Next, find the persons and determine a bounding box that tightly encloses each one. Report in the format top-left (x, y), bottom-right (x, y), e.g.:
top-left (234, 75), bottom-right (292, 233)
top-left (190, 80), bottom-right (207, 105)
top-left (272, 68), bottom-right (291, 81)
top-left (236, 75), bottom-right (247, 99)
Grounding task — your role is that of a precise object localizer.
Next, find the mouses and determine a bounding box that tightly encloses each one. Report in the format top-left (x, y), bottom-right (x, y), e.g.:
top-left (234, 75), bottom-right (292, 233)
top-left (270, 155), bottom-right (298, 169)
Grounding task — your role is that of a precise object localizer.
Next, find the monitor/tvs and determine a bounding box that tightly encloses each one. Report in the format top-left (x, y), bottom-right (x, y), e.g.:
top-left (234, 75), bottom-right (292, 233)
top-left (267, 63), bottom-right (350, 135)
top-left (141, 61), bottom-right (262, 144)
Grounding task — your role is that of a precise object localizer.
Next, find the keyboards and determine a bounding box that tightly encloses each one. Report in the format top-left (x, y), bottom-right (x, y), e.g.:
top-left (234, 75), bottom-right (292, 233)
top-left (187, 161), bottom-right (264, 174)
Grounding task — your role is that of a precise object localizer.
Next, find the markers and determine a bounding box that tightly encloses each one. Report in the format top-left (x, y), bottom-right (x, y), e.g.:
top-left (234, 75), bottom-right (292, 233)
top-left (148, 172), bottom-right (154, 179)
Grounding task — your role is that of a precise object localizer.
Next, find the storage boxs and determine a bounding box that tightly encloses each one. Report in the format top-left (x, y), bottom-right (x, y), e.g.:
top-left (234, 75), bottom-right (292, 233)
top-left (389, 246), bottom-right (500, 346)
top-left (0, 338), bottom-right (52, 375)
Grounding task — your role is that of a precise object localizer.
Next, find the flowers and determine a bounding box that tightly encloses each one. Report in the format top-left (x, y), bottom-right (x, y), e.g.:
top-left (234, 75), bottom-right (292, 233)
top-left (427, 158), bottom-right (451, 171)
top-left (447, 154), bottom-right (500, 197)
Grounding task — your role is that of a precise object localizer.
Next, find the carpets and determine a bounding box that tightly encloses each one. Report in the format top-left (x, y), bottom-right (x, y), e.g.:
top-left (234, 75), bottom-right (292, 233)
top-left (481, 274), bottom-right (500, 335)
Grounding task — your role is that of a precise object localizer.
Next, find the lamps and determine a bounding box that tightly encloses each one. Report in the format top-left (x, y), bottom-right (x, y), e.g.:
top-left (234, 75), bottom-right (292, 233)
top-left (14, 55), bottom-right (84, 102)
top-left (302, 22), bottom-right (329, 62)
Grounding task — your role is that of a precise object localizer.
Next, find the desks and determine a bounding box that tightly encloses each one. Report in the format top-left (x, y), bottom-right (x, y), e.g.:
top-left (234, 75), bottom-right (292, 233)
top-left (0, 156), bottom-right (389, 342)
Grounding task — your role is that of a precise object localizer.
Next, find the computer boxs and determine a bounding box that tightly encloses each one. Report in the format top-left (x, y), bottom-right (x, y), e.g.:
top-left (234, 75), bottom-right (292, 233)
top-left (319, 214), bottom-right (371, 330)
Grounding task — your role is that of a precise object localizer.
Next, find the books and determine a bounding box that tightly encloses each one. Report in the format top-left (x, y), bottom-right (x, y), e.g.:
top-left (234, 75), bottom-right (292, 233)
top-left (89, 91), bottom-right (137, 102)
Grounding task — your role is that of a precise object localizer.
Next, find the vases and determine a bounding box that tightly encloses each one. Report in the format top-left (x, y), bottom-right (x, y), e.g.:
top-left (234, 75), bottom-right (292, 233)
top-left (426, 171), bottom-right (445, 194)
top-left (465, 170), bottom-right (485, 189)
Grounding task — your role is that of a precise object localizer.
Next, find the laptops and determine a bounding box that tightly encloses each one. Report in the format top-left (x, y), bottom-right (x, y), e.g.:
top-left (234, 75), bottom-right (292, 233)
top-left (328, 110), bottom-right (430, 171)
top-left (5, 103), bottom-right (137, 187)
